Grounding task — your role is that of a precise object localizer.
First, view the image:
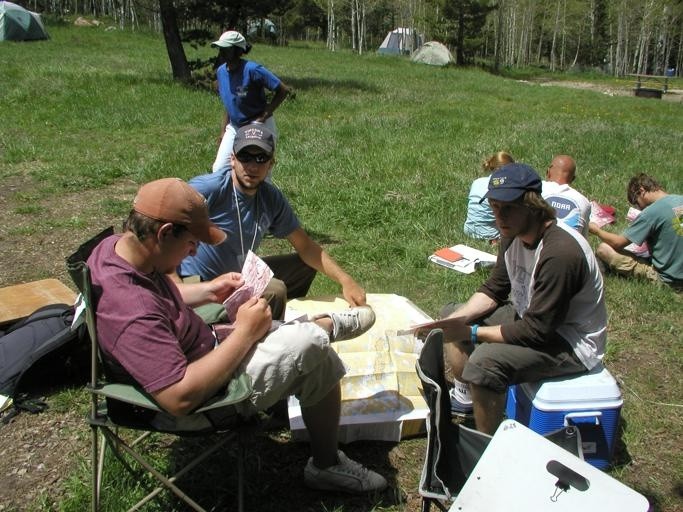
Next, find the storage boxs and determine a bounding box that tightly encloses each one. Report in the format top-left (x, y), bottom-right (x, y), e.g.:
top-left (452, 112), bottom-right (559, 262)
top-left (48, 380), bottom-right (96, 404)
top-left (503, 364), bottom-right (624, 472)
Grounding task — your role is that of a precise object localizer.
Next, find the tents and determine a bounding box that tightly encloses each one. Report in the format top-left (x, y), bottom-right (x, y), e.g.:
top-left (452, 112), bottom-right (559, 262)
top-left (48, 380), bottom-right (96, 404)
top-left (247, 17), bottom-right (276, 39)
top-left (377, 26), bottom-right (422, 54)
top-left (0, 1), bottom-right (49, 41)
top-left (410, 41), bottom-right (457, 67)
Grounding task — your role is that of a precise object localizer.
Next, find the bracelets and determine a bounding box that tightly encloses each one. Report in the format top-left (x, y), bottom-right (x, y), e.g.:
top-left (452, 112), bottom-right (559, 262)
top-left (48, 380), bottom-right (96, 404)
top-left (470, 324), bottom-right (478, 343)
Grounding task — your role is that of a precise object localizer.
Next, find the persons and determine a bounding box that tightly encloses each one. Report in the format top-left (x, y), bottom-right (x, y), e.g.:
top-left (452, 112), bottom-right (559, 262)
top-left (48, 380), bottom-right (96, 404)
top-left (178, 124), bottom-right (367, 319)
top-left (464, 151), bottom-right (514, 243)
top-left (88, 174), bottom-right (388, 495)
top-left (419, 166), bottom-right (606, 436)
top-left (212, 29), bottom-right (287, 183)
top-left (539, 155), bottom-right (590, 238)
top-left (589, 173), bottom-right (682, 295)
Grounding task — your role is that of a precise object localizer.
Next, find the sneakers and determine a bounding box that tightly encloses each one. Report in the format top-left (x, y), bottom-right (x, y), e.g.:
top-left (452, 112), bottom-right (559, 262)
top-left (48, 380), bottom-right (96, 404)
top-left (308, 304), bottom-right (376, 344)
top-left (304, 449), bottom-right (388, 495)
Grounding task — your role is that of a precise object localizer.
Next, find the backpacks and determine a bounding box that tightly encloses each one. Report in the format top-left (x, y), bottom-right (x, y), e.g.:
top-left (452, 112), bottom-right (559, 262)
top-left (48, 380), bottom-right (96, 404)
top-left (0, 303), bottom-right (92, 424)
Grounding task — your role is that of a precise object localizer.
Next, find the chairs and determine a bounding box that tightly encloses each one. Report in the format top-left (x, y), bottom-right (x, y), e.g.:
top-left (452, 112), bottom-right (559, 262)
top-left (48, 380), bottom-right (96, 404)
top-left (64, 227), bottom-right (257, 510)
top-left (412, 330), bottom-right (583, 511)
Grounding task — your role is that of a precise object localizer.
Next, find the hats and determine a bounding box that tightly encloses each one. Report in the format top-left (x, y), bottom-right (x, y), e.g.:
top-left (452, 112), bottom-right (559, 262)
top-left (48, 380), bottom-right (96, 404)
top-left (210, 31), bottom-right (247, 53)
top-left (235, 122), bottom-right (277, 158)
top-left (132, 177), bottom-right (228, 248)
top-left (479, 163), bottom-right (542, 205)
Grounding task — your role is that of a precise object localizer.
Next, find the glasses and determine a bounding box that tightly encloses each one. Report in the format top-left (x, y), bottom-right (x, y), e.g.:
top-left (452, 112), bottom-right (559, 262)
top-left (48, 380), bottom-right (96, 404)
top-left (235, 151), bottom-right (269, 164)
